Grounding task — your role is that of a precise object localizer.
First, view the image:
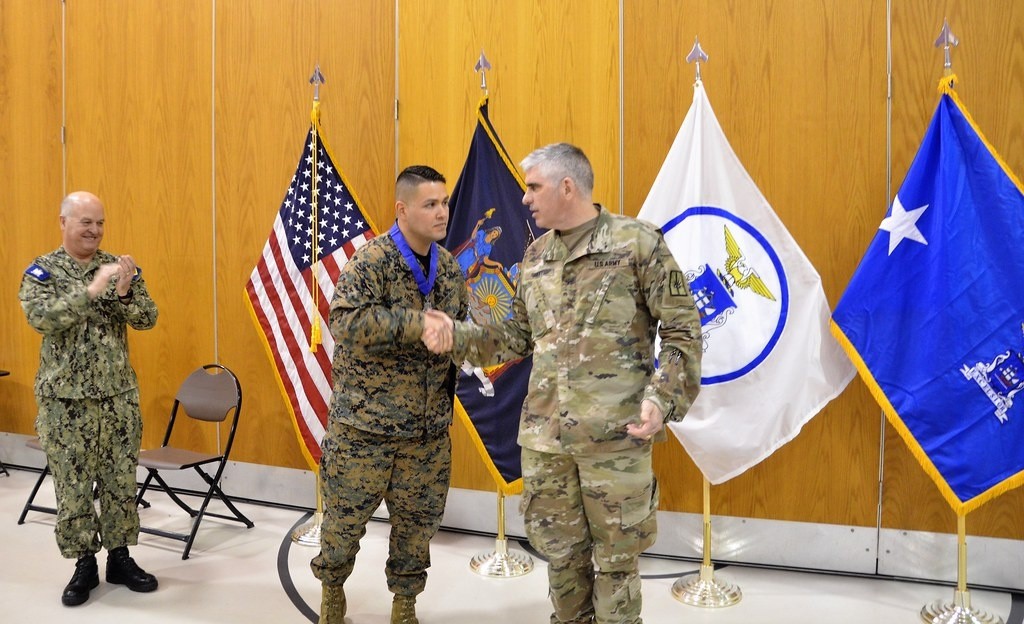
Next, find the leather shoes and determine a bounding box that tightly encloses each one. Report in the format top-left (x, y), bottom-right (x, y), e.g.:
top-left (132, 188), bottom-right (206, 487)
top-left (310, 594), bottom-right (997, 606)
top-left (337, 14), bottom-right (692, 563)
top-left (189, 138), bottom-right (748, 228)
top-left (105, 550), bottom-right (158, 592)
top-left (62, 556), bottom-right (100, 606)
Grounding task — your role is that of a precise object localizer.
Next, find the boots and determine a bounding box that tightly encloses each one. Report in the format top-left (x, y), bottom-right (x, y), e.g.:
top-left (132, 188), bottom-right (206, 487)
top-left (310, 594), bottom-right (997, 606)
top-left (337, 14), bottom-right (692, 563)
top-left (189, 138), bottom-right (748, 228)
top-left (390, 594), bottom-right (419, 624)
top-left (319, 583), bottom-right (346, 624)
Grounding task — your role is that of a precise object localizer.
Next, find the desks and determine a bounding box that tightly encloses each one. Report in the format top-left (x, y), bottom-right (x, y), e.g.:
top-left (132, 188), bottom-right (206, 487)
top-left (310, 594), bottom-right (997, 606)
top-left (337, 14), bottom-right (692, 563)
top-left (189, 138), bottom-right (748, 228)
top-left (0, 370), bottom-right (10, 476)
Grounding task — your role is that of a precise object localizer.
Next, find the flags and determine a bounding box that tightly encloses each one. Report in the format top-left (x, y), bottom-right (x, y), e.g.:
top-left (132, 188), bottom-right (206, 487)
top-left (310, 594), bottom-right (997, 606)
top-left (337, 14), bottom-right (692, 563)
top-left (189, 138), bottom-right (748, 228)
top-left (829, 78), bottom-right (1024, 515)
top-left (637, 82), bottom-right (856, 484)
top-left (246, 98), bottom-right (379, 470)
top-left (435, 97), bottom-right (550, 496)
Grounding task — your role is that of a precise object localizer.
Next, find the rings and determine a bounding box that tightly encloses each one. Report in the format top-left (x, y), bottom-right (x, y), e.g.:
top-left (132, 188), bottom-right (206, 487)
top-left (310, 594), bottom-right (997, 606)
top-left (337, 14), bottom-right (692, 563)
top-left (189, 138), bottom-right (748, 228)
top-left (646, 434), bottom-right (651, 440)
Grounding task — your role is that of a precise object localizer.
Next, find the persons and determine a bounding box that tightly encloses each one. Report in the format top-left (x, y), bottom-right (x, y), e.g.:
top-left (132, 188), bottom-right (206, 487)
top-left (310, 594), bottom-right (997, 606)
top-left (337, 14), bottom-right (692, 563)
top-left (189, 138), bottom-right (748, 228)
top-left (311, 166), bottom-right (468, 623)
top-left (421, 143), bottom-right (702, 624)
top-left (17, 191), bottom-right (158, 606)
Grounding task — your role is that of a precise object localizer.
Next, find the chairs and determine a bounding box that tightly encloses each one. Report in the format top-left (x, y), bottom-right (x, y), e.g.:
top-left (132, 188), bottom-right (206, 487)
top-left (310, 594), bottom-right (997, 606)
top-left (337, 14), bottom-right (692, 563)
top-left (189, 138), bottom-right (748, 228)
top-left (133, 364), bottom-right (254, 560)
top-left (19, 440), bottom-right (150, 524)
